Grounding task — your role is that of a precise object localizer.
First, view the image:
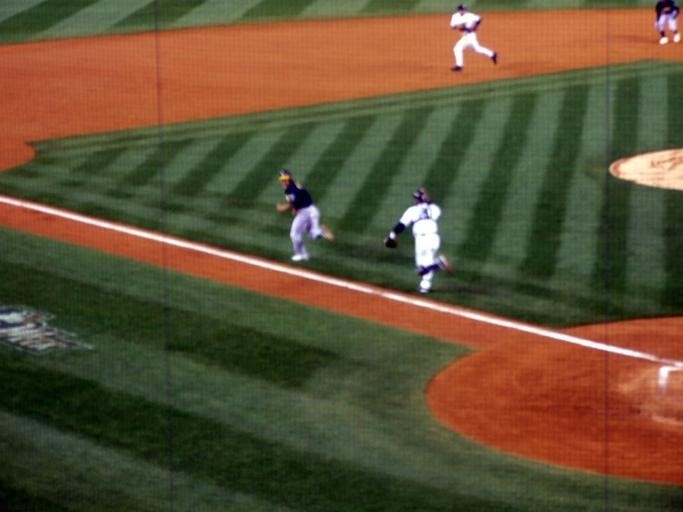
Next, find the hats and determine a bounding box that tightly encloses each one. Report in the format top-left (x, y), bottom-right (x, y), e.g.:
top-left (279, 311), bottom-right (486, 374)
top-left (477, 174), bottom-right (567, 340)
top-left (458, 5), bottom-right (465, 10)
top-left (278, 168), bottom-right (291, 180)
top-left (412, 187), bottom-right (429, 202)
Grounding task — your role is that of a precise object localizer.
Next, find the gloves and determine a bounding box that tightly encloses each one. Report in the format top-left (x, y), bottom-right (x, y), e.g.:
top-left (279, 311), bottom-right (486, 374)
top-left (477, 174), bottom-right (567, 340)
top-left (383, 236), bottom-right (399, 248)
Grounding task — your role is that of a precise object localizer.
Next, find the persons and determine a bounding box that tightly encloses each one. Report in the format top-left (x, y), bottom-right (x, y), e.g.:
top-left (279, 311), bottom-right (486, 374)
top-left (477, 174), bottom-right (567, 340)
top-left (654, 1), bottom-right (682, 46)
top-left (274, 167), bottom-right (334, 262)
top-left (382, 188), bottom-right (453, 295)
top-left (447, 4), bottom-right (498, 73)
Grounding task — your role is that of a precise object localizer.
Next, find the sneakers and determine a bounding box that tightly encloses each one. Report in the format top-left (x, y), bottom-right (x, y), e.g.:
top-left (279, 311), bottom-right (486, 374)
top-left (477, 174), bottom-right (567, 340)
top-left (416, 286), bottom-right (429, 294)
top-left (291, 254), bottom-right (308, 262)
top-left (660, 36), bottom-right (668, 44)
top-left (673, 33), bottom-right (681, 42)
top-left (439, 255), bottom-right (452, 273)
top-left (492, 52), bottom-right (497, 62)
top-left (320, 224), bottom-right (334, 241)
top-left (451, 66), bottom-right (461, 70)
